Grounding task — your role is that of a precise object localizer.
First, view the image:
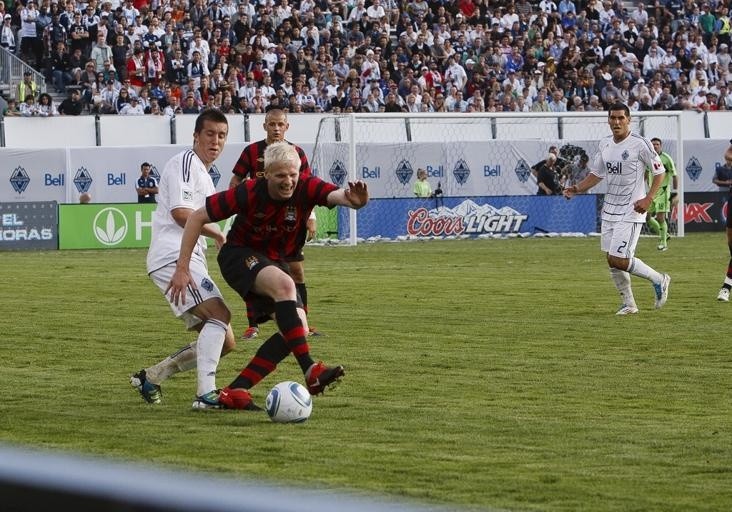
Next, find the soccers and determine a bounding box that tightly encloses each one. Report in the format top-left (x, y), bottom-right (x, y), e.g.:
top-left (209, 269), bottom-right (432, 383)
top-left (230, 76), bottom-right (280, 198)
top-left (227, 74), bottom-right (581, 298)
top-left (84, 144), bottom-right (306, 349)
top-left (266, 381), bottom-right (313, 424)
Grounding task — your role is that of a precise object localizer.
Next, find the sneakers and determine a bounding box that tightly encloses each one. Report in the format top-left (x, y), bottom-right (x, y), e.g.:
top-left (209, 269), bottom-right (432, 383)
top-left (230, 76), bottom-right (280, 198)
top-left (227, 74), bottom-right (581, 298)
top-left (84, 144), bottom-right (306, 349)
top-left (656, 232), bottom-right (672, 252)
top-left (240, 326), bottom-right (261, 340)
top-left (128, 367), bottom-right (163, 406)
top-left (191, 388), bottom-right (225, 411)
top-left (307, 361), bottom-right (346, 397)
top-left (614, 303), bottom-right (639, 317)
top-left (308, 325), bottom-right (329, 337)
top-left (218, 384), bottom-right (266, 413)
top-left (650, 272), bottom-right (672, 310)
top-left (715, 286), bottom-right (731, 302)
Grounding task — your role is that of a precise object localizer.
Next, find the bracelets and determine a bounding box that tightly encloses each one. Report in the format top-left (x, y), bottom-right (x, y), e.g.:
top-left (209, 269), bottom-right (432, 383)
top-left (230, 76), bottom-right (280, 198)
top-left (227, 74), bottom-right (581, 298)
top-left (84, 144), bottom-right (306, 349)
top-left (309, 211), bottom-right (316, 220)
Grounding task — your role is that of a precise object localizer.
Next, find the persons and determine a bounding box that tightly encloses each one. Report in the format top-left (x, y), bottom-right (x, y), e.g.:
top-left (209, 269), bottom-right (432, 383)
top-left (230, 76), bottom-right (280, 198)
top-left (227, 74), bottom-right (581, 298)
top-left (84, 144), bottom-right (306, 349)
top-left (129, 110), bottom-right (236, 411)
top-left (164, 140), bottom-right (369, 411)
top-left (644, 137), bottom-right (679, 252)
top-left (414, 168), bottom-right (432, 197)
top-left (530, 147), bottom-right (591, 194)
top-left (135, 162), bottom-right (159, 203)
top-left (216, 109), bottom-right (317, 340)
top-left (711, 139), bottom-right (732, 301)
top-left (560, 102), bottom-right (671, 314)
top-left (1, 0), bottom-right (732, 116)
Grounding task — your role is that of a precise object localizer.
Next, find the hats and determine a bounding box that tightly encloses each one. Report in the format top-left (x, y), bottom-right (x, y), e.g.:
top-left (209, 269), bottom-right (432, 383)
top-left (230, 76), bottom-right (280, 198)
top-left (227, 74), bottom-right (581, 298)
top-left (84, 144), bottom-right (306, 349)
top-left (3, 0), bottom-right (726, 103)
